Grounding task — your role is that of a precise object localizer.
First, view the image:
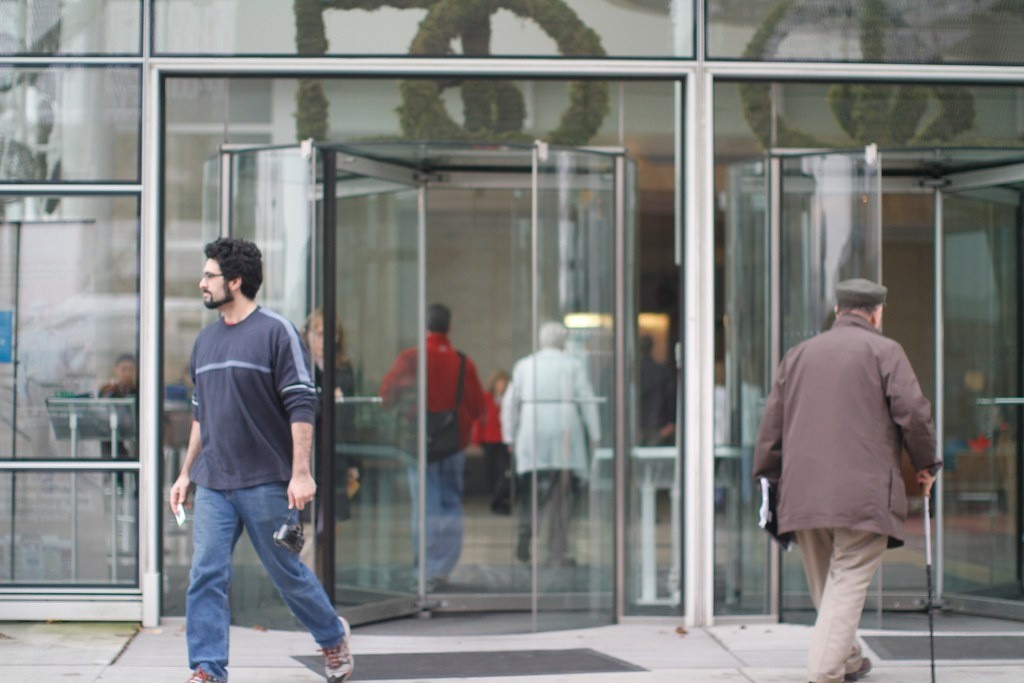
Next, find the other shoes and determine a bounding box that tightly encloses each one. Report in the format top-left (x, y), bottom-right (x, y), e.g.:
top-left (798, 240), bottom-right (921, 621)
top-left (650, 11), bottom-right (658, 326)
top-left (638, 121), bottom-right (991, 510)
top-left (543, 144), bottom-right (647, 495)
top-left (512, 526), bottom-right (531, 562)
top-left (546, 557), bottom-right (580, 573)
top-left (846, 657), bottom-right (872, 682)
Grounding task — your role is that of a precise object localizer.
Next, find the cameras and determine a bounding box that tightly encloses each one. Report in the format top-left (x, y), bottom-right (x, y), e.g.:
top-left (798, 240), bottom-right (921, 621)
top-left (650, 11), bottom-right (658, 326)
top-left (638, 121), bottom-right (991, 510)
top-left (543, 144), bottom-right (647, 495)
top-left (273, 524), bottom-right (306, 553)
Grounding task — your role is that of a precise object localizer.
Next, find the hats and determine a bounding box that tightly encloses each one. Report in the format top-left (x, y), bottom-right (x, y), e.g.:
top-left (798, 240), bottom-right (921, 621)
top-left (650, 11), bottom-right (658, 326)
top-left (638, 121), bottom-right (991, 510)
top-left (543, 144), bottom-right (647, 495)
top-left (836, 278), bottom-right (888, 306)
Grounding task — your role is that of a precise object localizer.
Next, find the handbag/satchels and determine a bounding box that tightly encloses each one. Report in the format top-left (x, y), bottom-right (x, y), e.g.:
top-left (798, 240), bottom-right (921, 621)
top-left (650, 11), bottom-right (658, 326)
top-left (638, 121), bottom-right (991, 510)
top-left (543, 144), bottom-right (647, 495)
top-left (404, 407), bottom-right (461, 463)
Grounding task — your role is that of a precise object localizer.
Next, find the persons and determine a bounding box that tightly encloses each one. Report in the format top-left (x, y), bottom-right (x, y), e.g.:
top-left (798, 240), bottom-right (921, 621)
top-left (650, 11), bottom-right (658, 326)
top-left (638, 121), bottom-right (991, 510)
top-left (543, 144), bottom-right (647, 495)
top-left (99, 353), bottom-right (138, 495)
top-left (638, 334), bottom-right (675, 444)
top-left (499, 320), bottom-right (602, 562)
top-left (473, 368), bottom-right (520, 514)
top-left (751, 279), bottom-right (943, 683)
top-left (169, 237), bottom-right (353, 683)
top-left (380, 303), bottom-right (486, 594)
top-left (303, 305), bottom-right (360, 521)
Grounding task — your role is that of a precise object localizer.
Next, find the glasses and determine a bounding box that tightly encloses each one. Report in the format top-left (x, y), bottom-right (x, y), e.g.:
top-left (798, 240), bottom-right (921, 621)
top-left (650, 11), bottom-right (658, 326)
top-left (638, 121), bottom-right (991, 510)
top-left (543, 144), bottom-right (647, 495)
top-left (201, 272), bottom-right (225, 279)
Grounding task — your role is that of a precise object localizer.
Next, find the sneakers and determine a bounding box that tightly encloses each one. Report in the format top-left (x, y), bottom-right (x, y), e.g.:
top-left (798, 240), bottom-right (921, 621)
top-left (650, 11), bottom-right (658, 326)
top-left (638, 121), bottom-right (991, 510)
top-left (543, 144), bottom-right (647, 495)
top-left (187, 667), bottom-right (231, 683)
top-left (322, 618), bottom-right (355, 682)
